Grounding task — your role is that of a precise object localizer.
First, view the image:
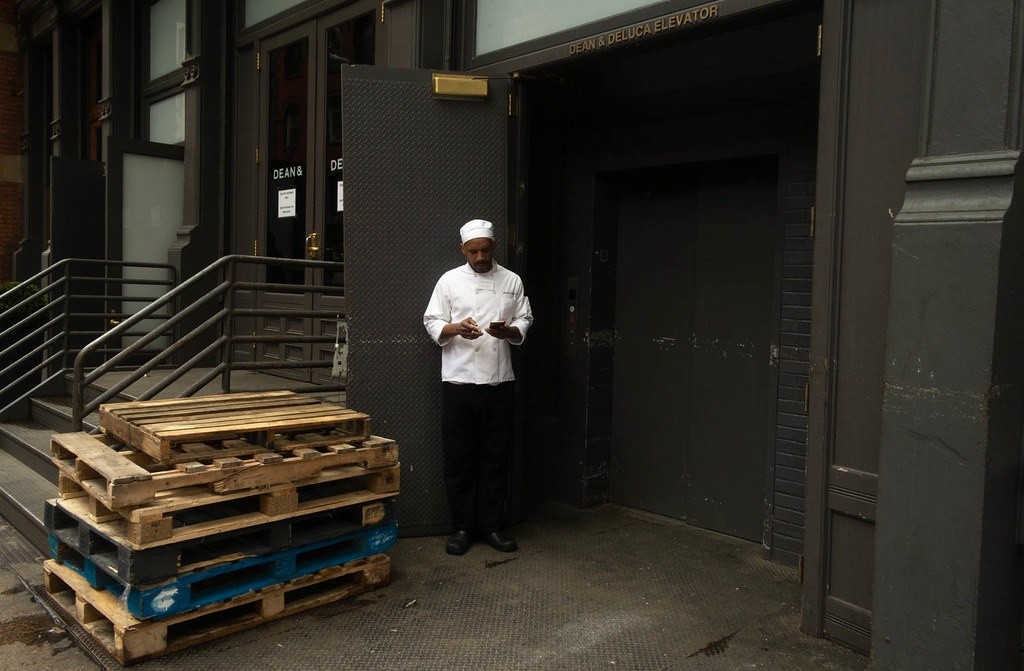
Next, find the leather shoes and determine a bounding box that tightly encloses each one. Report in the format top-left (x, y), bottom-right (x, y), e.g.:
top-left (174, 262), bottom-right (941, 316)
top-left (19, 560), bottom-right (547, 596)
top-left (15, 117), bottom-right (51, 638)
top-left (446, 530), bottom-right (472, 555)
top-left (477, 530), bottom-right (517, 552)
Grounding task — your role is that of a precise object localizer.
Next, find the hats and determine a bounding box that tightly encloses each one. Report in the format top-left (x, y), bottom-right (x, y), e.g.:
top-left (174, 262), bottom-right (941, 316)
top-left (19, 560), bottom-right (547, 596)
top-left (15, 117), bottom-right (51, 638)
top-left (460, 219), bottom-right (494, 244)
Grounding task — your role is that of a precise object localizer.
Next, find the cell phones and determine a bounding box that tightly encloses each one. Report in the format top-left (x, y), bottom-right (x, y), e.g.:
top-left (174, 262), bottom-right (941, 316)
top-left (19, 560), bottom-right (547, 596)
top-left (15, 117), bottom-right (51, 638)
top-left (489, 320), bottom-right (506, 330)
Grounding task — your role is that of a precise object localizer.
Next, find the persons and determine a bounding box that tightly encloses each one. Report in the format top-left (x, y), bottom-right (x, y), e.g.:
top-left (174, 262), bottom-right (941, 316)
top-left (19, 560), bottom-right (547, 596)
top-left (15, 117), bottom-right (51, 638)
top-left (423, 219), bottom-right (533, 555)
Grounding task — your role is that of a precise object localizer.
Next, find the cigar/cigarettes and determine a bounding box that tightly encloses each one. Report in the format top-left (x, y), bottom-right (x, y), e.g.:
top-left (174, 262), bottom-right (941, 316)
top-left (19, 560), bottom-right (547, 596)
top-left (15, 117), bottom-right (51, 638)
top-left (471, 324), bottom-right (475, 334)
